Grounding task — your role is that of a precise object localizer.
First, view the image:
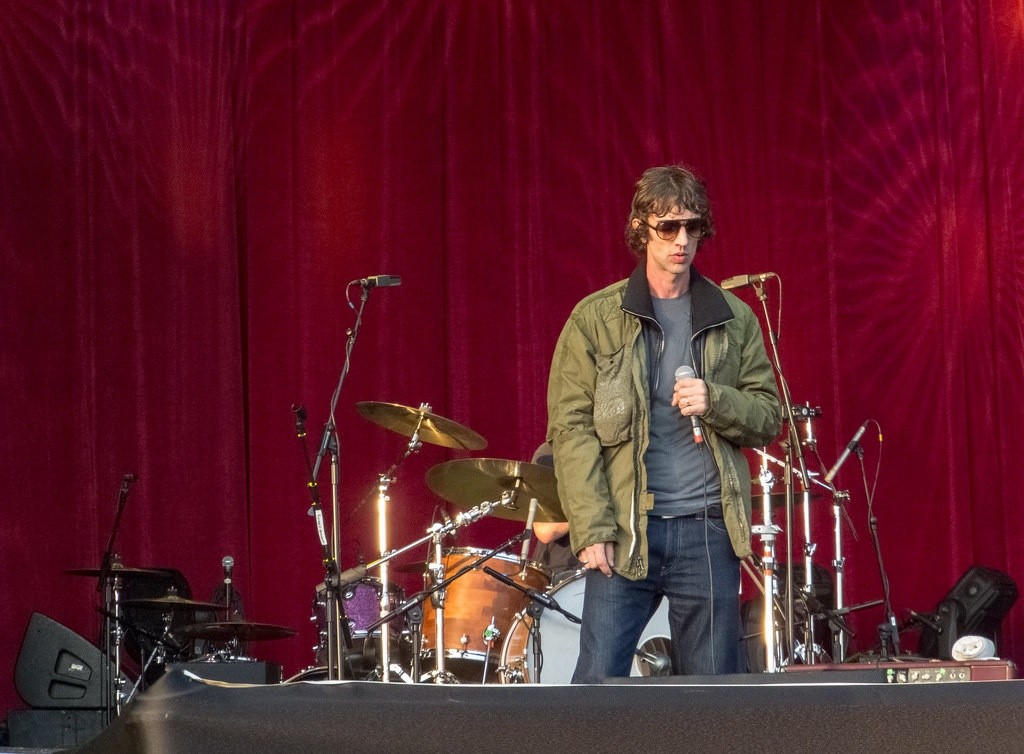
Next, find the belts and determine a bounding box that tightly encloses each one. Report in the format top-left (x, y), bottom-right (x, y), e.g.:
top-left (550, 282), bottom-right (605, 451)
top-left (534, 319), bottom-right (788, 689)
top-left (648, 507), bottom-right (723, 519)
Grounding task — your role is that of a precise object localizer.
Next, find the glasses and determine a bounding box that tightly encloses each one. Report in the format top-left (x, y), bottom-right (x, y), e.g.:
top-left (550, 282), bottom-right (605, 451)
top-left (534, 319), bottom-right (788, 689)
top-left (639, 217), bottom-right (708, 240)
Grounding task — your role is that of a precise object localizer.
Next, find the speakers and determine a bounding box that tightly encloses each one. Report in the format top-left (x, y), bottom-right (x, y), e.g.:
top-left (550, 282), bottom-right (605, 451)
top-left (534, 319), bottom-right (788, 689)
top-left (167, 661), bottom-right (280, 684)
top-left (14, 611), bottom-right (140, 708)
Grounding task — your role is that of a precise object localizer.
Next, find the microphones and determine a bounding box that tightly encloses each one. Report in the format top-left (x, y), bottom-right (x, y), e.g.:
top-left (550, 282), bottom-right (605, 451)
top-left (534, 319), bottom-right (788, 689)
top-left (651, 653), bottom-right (671, 672)
top-left (122, 474), bottom-right (137, 483)
top-left (720, 272), bottom-right (775, 290)
top-left (351, 275), bottom-right (401, 286)
top-left (439, 506), bottom-right (456, 536)
top-left (519, 497), bottom-right (539, 569)
top-left (906, 610), bottom-right (943, 634)
top-left (222, 555), bottom-right (235, 621)
top-left (825, 421), bottom-right (868, 482)
top-left (675, 365), bottom-right (703, 449)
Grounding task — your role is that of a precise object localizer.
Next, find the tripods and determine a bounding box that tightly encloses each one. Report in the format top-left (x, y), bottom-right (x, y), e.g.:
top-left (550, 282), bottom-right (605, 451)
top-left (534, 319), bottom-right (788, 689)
top-left (349, 416), bottom-right (524, 686)
top-left (752, 446), bottom-right (834, 668)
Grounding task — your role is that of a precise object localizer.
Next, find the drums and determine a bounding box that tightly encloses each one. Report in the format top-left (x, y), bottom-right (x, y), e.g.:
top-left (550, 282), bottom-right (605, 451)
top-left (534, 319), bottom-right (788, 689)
top-left (314, 577), bottom-right (405, 666)
top-left (497, 566), bottom-right (671, 685)
top-left (418, 547), bottom-right (553, 683)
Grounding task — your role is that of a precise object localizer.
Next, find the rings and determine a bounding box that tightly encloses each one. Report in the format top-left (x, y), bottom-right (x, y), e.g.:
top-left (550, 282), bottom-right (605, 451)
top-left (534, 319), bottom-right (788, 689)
top-left (582, 560), bottom-right (589, 565)
top-left (685, 396), bottom-right (690, 406)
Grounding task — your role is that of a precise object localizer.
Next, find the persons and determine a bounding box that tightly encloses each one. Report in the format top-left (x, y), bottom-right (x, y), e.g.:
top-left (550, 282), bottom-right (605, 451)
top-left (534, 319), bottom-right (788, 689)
top-left (548, 161), bottom-right (781, 687)
top-left (532, 443), bottom-right (579, 573)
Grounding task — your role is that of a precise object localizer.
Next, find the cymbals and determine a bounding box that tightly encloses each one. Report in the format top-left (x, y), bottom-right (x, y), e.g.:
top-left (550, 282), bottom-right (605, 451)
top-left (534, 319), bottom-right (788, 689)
top-left (354, 401), bottom-right (488, 451)
top-left (425, 457), bottom-right (568, 523)
top-left (173, 622), bottom-right (300, 641)
top-left (115, 595), bottom-right (228, 611)
top-left (64, 563), bottom-right (170, 580)
top-left (751, 492), bottom-right (823, 509)
top-left (393, 559), bottom-right (426, 572)
